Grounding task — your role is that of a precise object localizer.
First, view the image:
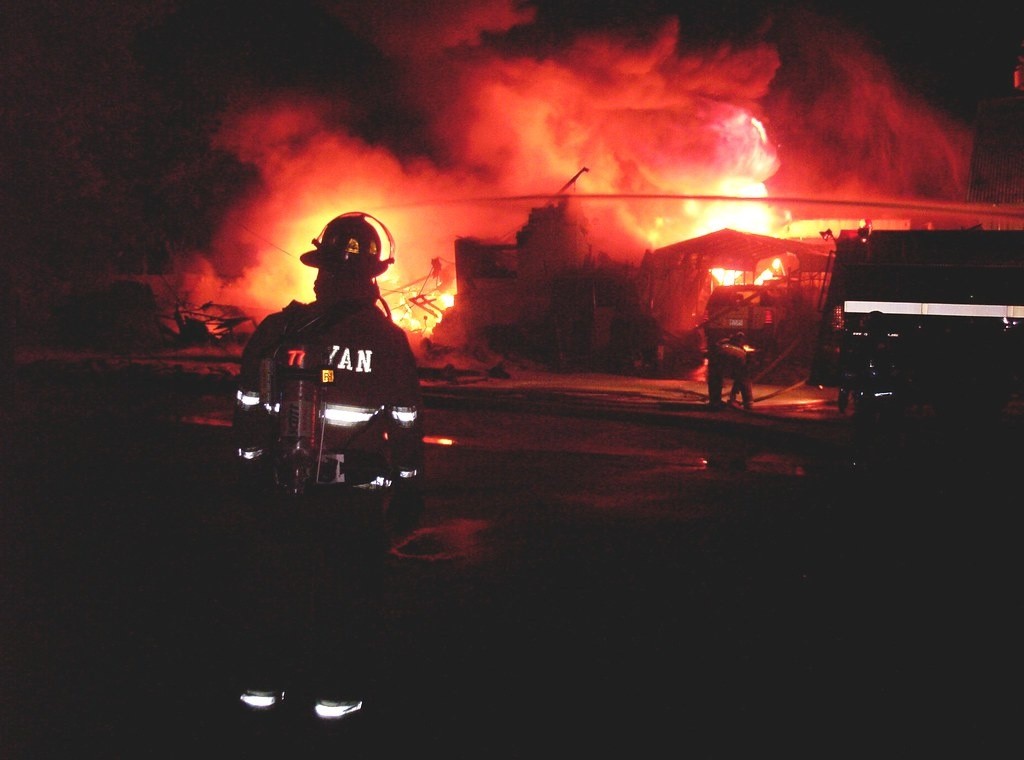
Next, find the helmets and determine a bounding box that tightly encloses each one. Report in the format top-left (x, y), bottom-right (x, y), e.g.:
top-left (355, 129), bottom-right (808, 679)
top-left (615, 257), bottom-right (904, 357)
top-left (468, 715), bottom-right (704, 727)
top-left (300, 211), bottom-right (394, 277)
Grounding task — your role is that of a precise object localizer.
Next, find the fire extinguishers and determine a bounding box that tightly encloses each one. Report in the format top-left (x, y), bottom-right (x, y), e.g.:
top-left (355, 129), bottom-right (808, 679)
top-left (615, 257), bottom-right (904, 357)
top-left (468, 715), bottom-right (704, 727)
top-left (275, 343), bottom-right (318, 504)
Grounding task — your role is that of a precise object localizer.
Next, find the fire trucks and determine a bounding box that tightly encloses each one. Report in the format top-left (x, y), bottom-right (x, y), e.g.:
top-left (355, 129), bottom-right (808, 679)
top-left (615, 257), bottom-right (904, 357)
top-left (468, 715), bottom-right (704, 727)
top-left (809, 228), bottom-right (1024, 440)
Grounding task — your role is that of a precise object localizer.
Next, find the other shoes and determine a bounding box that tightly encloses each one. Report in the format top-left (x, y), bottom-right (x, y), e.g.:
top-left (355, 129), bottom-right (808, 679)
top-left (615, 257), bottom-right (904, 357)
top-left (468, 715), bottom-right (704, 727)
top-left (744, 403), bottom-right (754, 410)
top-left (711, 400), bottom-right (721, 407)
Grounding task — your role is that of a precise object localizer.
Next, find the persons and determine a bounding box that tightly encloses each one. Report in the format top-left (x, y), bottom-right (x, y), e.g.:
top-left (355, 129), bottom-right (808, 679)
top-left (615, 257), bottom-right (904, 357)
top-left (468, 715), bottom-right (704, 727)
top-left (708, 332), bottom-right (759, 408)
top-left (226, 213), bottom-right (422, 720)
